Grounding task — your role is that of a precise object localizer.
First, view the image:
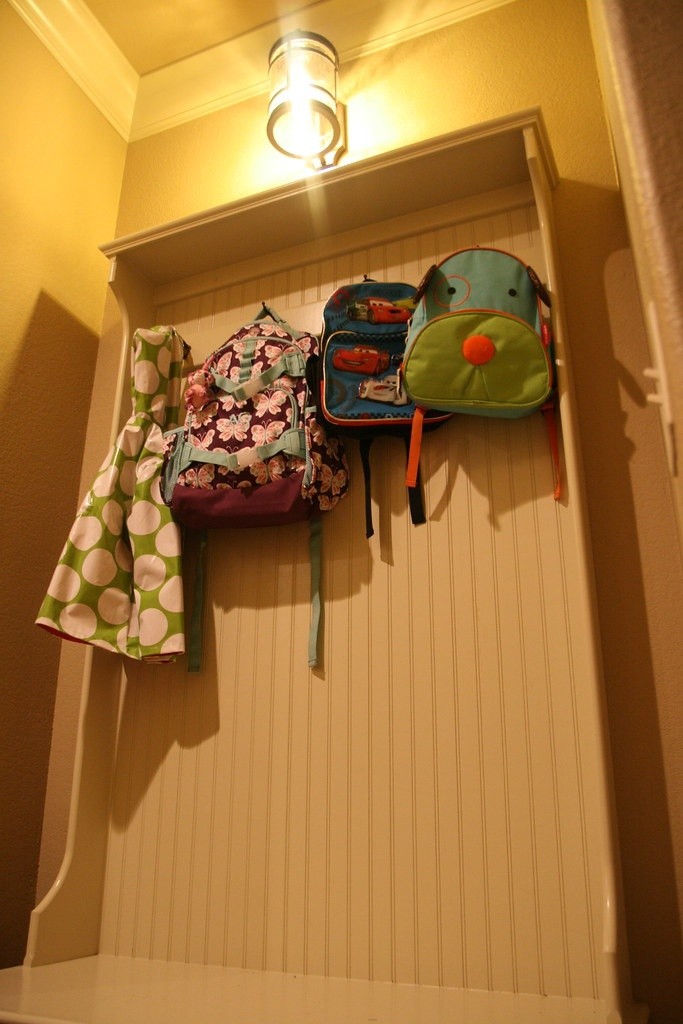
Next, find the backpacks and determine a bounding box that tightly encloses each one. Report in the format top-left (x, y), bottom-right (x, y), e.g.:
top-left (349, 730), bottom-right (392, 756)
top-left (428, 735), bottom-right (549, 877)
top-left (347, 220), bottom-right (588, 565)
top-left (395, 247), bottom-right (563, 420)
top-left (319, 277), bottom-right (462, 437)
top-left (162, 308), bottom-right (352, 530)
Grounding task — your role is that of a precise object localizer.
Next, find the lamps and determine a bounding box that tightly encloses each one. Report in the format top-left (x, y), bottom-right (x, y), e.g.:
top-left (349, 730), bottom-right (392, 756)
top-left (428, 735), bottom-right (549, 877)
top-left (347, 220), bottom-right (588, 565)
top-left (265, 30), bottom-right (347, 174)
